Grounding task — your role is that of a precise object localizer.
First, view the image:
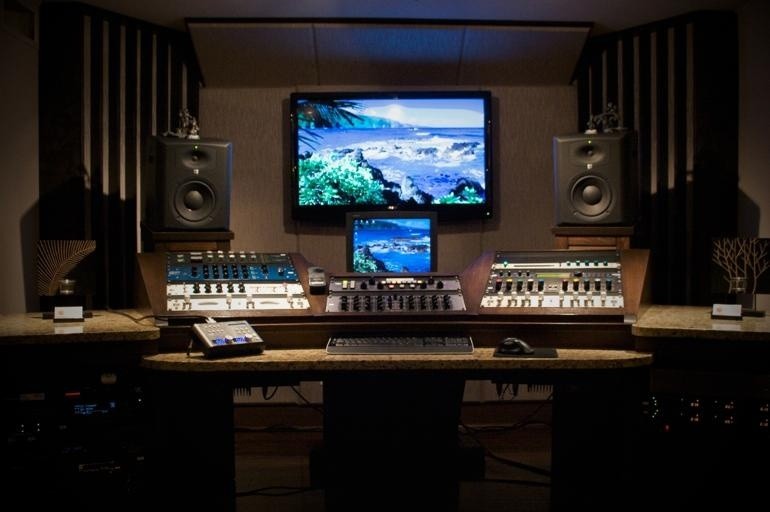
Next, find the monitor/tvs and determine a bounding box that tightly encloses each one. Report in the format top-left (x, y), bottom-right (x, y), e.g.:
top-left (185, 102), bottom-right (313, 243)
top-left (289, 90), bottom-right (492, 227)
top-left (346, 211), bottom-right (437, 272)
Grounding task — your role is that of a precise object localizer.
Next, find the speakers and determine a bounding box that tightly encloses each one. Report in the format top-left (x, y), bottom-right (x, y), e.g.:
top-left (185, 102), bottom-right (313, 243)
top-left (144, 136), bottom-right (231, 232)
top-left (553, 130), bottom-right (638, 225)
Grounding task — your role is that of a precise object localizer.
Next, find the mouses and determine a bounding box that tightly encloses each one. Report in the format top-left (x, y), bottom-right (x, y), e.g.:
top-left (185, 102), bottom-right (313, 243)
top-left (497, 338), bottom-right (535, 355)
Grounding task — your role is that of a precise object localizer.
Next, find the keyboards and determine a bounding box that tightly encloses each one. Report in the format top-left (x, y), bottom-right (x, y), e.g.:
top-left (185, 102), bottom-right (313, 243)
top-left (326, 335), bottom-right (474, 354)
